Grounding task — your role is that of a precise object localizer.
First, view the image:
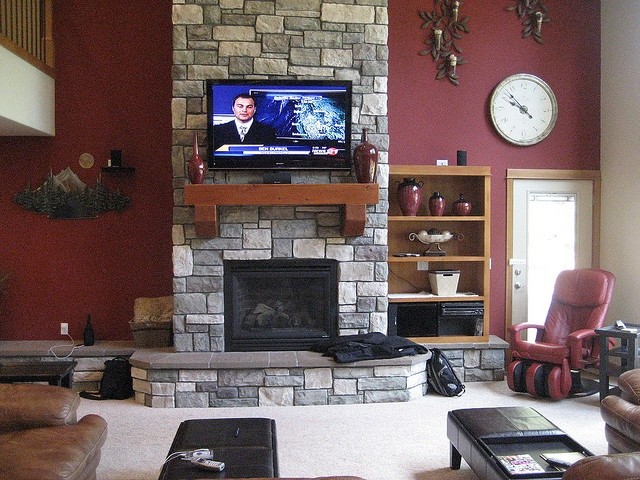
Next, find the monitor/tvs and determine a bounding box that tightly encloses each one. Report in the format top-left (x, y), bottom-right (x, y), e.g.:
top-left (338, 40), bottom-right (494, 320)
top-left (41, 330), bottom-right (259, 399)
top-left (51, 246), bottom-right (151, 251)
top-left (206, 79), bottom-right (352, 184)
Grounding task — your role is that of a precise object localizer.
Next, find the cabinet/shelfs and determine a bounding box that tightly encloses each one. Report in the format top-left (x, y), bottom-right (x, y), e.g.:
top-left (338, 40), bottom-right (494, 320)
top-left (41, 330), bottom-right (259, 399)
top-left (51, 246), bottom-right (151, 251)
top-left (594, 321), bottom-right (640, 404)
top-left (387, 163), bottom-right (491, 344)
top-left (182, 183), bottom-right (380, 240)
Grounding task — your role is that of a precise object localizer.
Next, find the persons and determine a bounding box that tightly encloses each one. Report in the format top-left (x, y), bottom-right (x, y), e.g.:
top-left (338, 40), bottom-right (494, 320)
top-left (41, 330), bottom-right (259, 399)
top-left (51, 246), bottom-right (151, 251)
top-left (213, 94), bottom-right (276, 150)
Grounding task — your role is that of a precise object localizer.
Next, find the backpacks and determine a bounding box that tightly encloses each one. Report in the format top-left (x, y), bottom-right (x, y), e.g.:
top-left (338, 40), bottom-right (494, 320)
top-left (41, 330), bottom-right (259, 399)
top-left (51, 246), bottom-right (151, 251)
top-left (427, 348), bottom-right (465, 397)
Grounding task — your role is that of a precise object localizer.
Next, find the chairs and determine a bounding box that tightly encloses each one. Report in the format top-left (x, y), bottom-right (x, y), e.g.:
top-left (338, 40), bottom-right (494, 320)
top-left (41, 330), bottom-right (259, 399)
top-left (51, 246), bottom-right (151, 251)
top-left (506, 266), bottom-right (617, 400)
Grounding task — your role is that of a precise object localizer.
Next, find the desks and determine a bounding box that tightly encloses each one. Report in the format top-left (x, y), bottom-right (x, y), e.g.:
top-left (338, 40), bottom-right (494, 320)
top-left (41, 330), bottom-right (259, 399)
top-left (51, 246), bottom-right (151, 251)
top-left (1, 361), bottom-right (78, 389)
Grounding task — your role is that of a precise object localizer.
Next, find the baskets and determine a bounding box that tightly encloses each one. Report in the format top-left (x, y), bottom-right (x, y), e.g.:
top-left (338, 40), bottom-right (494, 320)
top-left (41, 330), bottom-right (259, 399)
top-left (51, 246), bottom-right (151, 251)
top-left (128, 318), bottom-right (173, 348)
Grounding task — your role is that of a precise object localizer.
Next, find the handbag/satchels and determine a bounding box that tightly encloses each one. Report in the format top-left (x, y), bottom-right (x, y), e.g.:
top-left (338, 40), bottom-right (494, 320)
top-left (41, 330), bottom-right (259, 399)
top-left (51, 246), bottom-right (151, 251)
top-left (100, 357), bottom-right (135, 400)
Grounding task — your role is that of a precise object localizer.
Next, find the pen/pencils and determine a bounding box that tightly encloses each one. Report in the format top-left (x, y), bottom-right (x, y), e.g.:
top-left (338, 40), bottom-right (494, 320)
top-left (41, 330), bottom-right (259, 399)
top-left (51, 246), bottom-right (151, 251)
top-left (234, 427), bottom-right (240, 437)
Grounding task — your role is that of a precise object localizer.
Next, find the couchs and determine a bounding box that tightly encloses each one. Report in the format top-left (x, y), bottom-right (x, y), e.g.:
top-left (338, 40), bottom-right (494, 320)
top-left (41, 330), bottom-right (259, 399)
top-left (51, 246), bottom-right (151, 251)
top-left (563, 367), bottom-right (640, 479)
top-left (1, 382), bottom-right (110, 480)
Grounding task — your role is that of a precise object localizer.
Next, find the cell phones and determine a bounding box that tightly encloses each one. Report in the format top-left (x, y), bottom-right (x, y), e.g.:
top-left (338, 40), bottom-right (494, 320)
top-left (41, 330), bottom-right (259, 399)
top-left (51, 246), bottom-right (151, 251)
top-left (191, 456), bottom-right (225, 472)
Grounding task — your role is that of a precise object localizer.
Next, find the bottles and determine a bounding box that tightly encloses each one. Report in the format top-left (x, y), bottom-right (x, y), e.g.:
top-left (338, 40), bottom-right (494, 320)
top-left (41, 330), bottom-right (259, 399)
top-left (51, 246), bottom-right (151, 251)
top-left (83, 315), bottom-right (94, 346)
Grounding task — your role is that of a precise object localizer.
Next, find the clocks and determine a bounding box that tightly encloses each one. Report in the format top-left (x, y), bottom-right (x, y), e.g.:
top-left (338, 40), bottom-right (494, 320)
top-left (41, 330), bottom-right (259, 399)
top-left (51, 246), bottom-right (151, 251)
top-left (487, 73), bottom-right (558, 146)
